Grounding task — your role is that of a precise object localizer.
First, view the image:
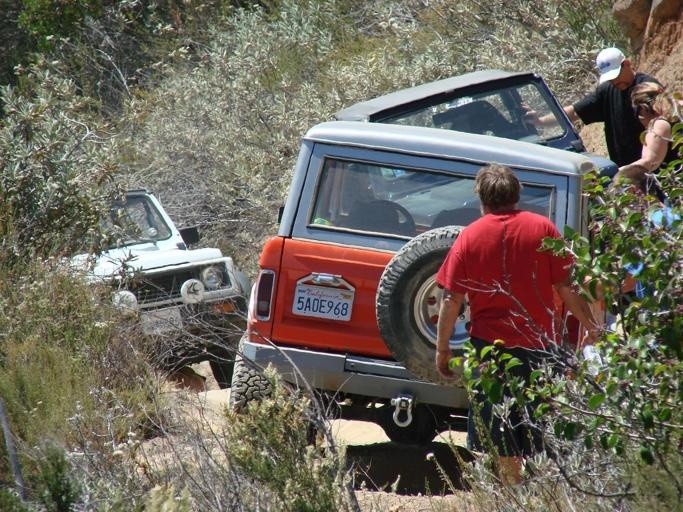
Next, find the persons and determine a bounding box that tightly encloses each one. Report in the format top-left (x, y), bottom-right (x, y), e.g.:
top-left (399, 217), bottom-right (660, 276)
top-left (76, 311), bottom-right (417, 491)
top-left (585, 166), bottom-right (683, 302)
top-left (522, 47), bottom-right (665, 166)
top-left (435, 164), bottom-right (601, 492)
top-left (613, 82), bottom-right (683, 208)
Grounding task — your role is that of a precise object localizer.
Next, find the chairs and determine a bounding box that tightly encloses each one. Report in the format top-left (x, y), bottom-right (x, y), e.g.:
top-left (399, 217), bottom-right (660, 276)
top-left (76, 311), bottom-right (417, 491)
top-left (345, 199), bottom-right (481, 244)
top-left (432, 100), bottom-right (529, 144)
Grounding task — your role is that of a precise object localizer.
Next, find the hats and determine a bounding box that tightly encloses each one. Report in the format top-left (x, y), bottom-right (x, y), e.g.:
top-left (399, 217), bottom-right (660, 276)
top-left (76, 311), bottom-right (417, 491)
top-left (595, 46), bottom-right (626, 87)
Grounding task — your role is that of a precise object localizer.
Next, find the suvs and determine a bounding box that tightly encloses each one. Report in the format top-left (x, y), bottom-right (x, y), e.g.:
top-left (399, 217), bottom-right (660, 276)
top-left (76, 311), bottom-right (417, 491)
top-left (229, 117), bottom-right (593, 452)
top-left (328, 67), bottom-right (681, 313)
top-left (36, 188), bottom-right (248, 388)
